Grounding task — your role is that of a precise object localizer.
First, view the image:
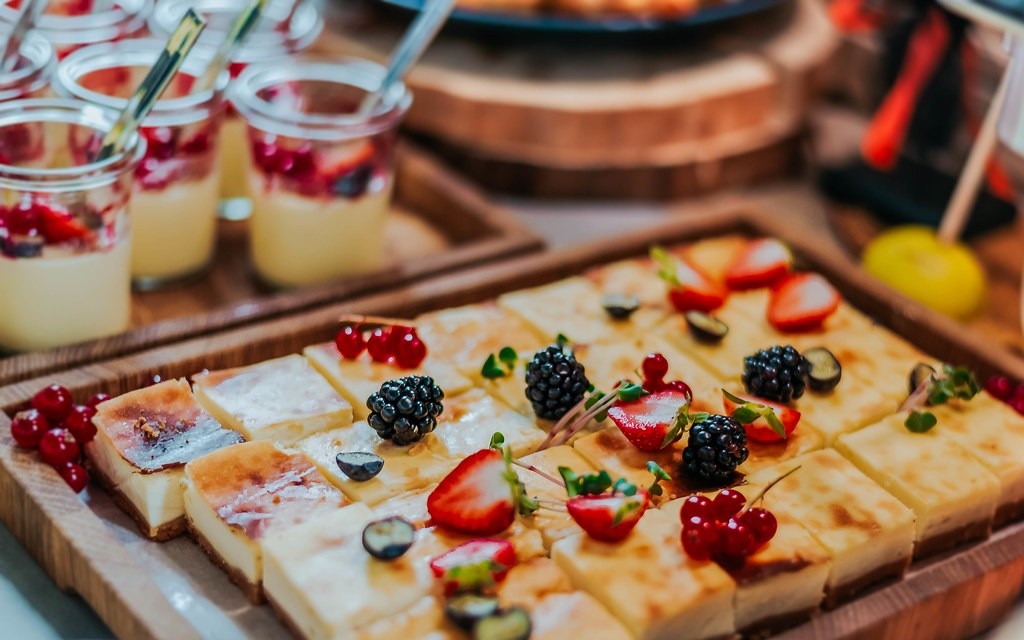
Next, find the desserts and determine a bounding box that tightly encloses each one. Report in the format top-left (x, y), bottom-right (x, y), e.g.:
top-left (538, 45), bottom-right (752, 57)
top-left (0, 0), bottom-right (413, 350)
top-left (83, 233), bottom-right (1024, 640)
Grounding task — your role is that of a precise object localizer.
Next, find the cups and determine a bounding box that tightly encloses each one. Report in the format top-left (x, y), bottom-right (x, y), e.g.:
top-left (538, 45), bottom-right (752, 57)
top-left (0, 0), bottom-right (414, 353)
